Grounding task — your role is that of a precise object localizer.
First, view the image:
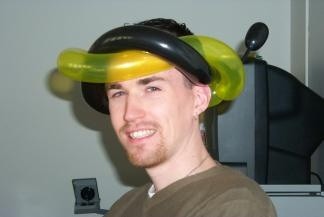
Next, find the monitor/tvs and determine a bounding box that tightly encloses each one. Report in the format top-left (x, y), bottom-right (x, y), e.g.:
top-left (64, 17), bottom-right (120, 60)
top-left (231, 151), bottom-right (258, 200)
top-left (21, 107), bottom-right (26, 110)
top-left (207, 59), bottom-right (324, 185)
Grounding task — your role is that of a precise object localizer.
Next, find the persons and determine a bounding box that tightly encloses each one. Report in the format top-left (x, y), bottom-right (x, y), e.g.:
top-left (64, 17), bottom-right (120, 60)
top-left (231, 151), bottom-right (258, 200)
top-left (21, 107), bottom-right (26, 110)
top-left (101, 16), bottom-right (278, 217)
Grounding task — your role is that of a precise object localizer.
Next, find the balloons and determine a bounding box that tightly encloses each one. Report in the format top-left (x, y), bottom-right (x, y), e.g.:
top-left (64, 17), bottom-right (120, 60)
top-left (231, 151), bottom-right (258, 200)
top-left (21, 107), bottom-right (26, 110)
top-left (57, 22), bottom-right (267, 116)
